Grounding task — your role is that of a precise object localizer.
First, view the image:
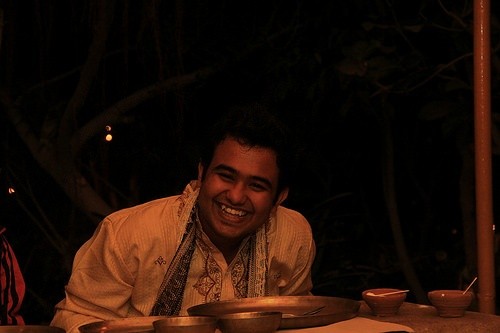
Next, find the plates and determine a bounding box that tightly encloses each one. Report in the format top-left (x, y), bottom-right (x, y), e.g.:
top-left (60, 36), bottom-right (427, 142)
top-left (187, 295), bottom-right (361, 329)
top-left (0, 325), bottom-right (67, 333)
top-left (78, 316), bottom-right (223, 333)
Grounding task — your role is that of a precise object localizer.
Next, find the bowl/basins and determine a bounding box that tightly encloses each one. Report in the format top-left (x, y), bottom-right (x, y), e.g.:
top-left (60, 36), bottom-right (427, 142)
top-left (152, 316), bottom-right (217, 333)
top-left (219, 310), bottom-right (282, 333)
top-left (361, 288), bottom-right (406, 316)
top-left (428, 290), bottom-right (475, 317)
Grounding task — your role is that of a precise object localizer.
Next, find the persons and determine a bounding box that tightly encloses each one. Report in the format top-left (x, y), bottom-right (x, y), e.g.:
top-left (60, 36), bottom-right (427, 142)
top-left (47, 104), bottom-right (317, 333)
